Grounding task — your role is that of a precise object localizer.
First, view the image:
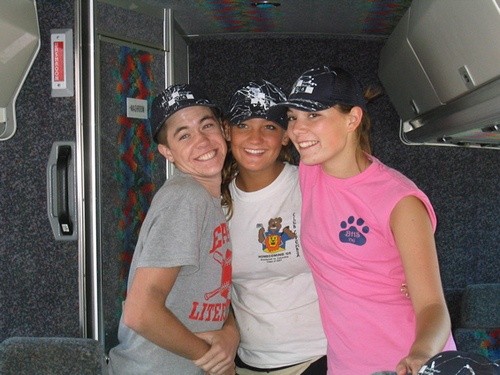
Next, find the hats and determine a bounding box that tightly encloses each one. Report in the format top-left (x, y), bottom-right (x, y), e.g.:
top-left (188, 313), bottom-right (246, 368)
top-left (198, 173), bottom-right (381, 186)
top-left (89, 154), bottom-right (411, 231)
top-left (283, 65), bottom-right (362, 111)
top-left (152, 83), bottom-right (218, 138)
top-left (226, 79), bottom-right (287, 129)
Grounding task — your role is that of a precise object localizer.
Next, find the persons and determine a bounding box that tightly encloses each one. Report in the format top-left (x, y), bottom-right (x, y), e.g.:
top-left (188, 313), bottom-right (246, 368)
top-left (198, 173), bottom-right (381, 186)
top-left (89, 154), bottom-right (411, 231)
top-left (220, 77), bottom-right (411, 375)
top-left (105, 83), bottom-right (240, 375)
top-left (266, 65), bottom-right (457, 375)
